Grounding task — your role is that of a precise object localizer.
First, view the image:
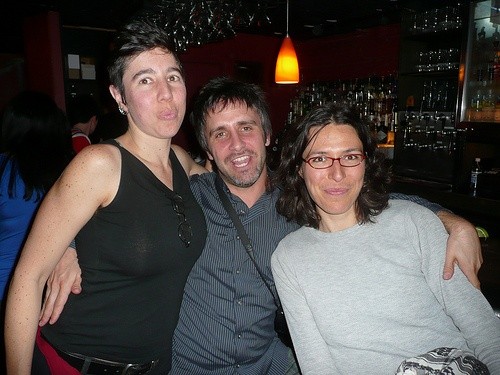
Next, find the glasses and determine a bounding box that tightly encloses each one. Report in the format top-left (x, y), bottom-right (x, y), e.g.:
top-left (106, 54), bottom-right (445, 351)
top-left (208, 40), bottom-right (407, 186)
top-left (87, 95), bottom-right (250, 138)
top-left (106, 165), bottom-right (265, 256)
top-left (301, 153), bottom-right (368, 170)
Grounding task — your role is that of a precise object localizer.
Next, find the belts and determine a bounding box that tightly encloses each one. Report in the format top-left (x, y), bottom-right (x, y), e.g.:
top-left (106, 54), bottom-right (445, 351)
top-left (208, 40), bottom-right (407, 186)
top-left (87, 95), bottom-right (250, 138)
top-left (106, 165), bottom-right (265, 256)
top-left (41, 332), bottom-right (161, 375)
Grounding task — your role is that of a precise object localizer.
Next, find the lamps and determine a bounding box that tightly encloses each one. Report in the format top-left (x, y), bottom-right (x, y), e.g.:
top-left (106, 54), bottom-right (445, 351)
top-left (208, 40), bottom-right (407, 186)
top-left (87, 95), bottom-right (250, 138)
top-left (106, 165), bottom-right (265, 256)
top-left (274, 0), bottom-right (299, 84)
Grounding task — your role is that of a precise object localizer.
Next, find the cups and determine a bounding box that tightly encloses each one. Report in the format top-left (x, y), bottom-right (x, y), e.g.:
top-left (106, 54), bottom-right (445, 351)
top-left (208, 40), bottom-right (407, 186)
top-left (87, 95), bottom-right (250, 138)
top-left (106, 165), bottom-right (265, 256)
top-left (407, 4), bottom-right (463, 30)
top-left (415, 46), bottom-right (459, 72)
top-left (421, 79), bottom-right (453, 111)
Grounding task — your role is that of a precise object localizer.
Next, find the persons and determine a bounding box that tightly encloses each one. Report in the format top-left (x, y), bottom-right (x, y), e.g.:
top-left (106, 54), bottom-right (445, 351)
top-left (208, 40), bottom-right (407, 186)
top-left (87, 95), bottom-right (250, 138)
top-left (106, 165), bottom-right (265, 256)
top-left (2, 22), bottom-right (213, 375)
top-left (61, 95), bottom-right (100, 160)
top-left (269, 103), bottom-right (500, 375)
top-left (39, 74), bottom-right (483, 375)
top-left (0, 93), bottom-right (81, 374)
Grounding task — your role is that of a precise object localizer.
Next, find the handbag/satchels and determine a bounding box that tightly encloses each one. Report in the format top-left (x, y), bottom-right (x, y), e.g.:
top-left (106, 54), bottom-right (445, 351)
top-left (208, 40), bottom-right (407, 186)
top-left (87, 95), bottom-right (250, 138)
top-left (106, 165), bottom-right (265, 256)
top-left (273, 304), bottom-right (303, 375)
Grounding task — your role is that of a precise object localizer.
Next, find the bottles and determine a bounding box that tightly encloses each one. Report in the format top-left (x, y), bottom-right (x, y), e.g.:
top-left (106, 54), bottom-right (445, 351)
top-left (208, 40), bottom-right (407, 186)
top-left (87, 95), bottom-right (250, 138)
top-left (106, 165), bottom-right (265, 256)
top-left (286, 72), bottom-right (394, 147)
top-left (469, 157), bottom-right (482, 189)
top-left (396, 112), bottom-right (457, 159)
top-left (470, 51), bottom-right (500, 121)
